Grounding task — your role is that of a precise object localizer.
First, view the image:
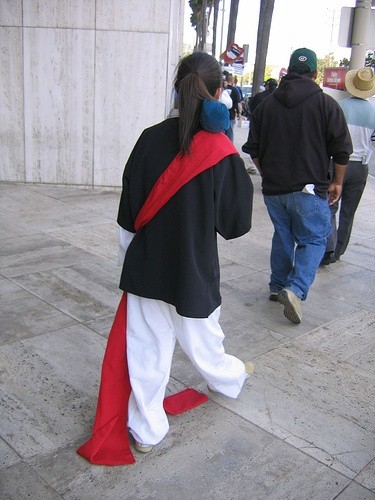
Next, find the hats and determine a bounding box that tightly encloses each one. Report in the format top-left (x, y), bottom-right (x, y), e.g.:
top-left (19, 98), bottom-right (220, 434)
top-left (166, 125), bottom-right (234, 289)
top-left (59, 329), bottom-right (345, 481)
top-left (344, 68), bottom-right (375, 98)
top-left (264, 78), bottom-right (277, 86)
top-left (289, 48), bottom-right (317, 74)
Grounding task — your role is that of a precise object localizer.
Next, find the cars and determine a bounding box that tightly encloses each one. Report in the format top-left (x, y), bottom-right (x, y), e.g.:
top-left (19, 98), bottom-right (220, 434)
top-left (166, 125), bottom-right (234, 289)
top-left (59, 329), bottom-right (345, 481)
top-left (237, 85), bottom-right (266, 105)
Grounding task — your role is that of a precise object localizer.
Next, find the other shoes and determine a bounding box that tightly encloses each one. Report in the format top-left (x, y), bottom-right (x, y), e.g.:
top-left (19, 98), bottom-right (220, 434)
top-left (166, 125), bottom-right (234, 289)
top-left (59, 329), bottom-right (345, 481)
top-left (320, 253), bottom-right (339, 265)
top-left (277, 288), bottom-right (303, 324)
top-left (269, 293), bottom-right (279, 301)
top-left (135, 440), bottom-right (153, 452)
top-left (205, 362), bottom-right (255, 392)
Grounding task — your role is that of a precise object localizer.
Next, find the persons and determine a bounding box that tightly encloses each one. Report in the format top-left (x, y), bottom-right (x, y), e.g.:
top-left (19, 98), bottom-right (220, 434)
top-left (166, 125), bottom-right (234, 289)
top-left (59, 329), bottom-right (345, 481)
top-left (246, 78), bottom-right (278, 174)
top-left (114, 51), bottom-right (255, 454)
top-left (219, 70), bottom-right (244, 144)
top-left (318, 67), bottom-right (375, 266)
top-left (242, 47), bottom-right (354, 324)
